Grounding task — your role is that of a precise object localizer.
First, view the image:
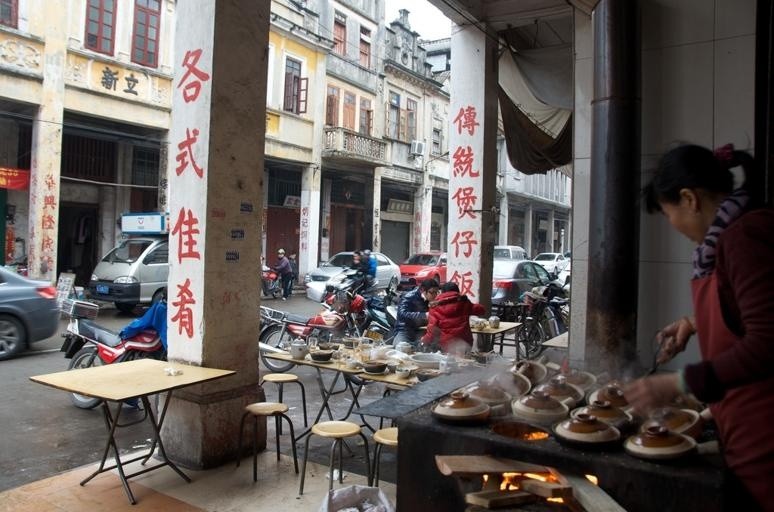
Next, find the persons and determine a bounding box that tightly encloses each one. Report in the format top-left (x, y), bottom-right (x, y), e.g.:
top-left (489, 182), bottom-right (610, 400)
top-left (271, 248), bottom-right (294, 300)
top-left (420, 277), bottom-right (487, 356)
top-left (615, 140), bottom-right (773, 510)
top-left (289, 251), bottom-right (299, 281)
top-left (361, 249), bottom-right (379, 283)
top-left (343, 248), bottom-right (367, 297)
top-left (393, 279), bottom-right (439, 350)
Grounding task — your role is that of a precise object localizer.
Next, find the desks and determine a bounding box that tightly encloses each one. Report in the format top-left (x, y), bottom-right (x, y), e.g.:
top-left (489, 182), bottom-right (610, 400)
top-left (358, 371), bottom-right (420, 430)
top-left (29, 359), bottom-right (236, 505)
top-left (542, 331), bottom-right (569, 348)
top-left (263, 351), bottom-right (388, 456)
top-left (418, 322), bottom-right (522, 352)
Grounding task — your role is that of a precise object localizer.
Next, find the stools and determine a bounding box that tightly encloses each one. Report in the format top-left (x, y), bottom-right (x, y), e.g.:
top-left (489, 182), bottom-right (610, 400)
top-left (300, 421), bottom-right (370, 496)
top-left (371, 427), bottom-right (398, 487)
top-left (260, 373), bottom-right (307, 435)
top-left (379, 385), bottom-right (410, 429)
top-left (237, 402), bottom-right (299, 482)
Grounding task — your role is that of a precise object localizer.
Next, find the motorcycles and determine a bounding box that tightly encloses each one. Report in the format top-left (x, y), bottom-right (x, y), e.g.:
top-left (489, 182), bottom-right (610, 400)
top-left (262, 268), bottom-right (284, 298)
top-left (260, 293), bottom-right (427, 374)
top-left (62, 297), bottom-right (168, 407)
top-left (515, 280), bottom-right (571, 357)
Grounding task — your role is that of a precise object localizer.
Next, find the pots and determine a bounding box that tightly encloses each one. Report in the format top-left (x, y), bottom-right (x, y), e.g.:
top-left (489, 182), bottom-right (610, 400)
top-left (622, 434), bottom-right (696, 460)
top-left (431, 358), bottom-right (701, 434)
top-left (549, 422), bottom-right (621, 448)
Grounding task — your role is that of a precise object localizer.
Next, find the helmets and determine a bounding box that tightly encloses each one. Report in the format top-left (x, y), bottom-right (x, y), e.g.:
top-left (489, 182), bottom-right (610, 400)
top-left (364, 250), bottom-right (371, 255)
top-left (277, 248), bottom-right (285, 254)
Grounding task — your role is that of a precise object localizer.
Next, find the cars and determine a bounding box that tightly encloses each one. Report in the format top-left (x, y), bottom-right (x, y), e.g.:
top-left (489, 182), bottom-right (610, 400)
top-left (0, 266), bottom-right (58, 362)
top-left (396, 251), bottom-right (448, 287)
top-left (533, 251), bottom-right (573, 283)
top-left (305, 249), bottom-right (402, 294)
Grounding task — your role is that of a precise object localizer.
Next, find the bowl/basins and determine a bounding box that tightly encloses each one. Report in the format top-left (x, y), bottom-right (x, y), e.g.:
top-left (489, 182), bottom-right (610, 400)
top-left (310, 336), bottom-right (441, 385)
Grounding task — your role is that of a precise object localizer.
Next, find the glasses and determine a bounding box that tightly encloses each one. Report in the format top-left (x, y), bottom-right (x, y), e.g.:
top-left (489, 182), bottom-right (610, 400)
top-left (428, 290), bottom-right (441, 296)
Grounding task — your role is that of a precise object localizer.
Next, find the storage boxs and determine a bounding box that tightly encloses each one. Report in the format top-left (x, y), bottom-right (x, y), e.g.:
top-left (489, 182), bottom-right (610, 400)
top-left (60, 298), bottom-right (99, 320)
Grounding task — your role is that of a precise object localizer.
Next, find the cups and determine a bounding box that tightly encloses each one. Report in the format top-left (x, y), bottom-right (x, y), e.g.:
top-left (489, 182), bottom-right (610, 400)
top-left (469, 315), bottom-right (487, 333)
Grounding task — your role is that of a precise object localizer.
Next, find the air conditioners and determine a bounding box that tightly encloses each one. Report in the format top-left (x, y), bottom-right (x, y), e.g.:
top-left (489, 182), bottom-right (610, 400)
top-left (411, 140), bottom-right (425, 156)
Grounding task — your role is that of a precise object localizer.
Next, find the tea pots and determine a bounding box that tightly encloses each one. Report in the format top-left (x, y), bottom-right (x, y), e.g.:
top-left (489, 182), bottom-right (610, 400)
top-left (287, 337), bottom-right (311, 360)
top-left (489, 315), bottom-right (499, 328)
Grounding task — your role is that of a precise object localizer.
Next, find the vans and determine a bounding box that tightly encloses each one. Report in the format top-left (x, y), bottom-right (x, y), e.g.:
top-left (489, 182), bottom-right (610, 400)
top-left (492, 247), bottom-right (529, 284)
top-left (88, 237), bottom-right (168, 306)
